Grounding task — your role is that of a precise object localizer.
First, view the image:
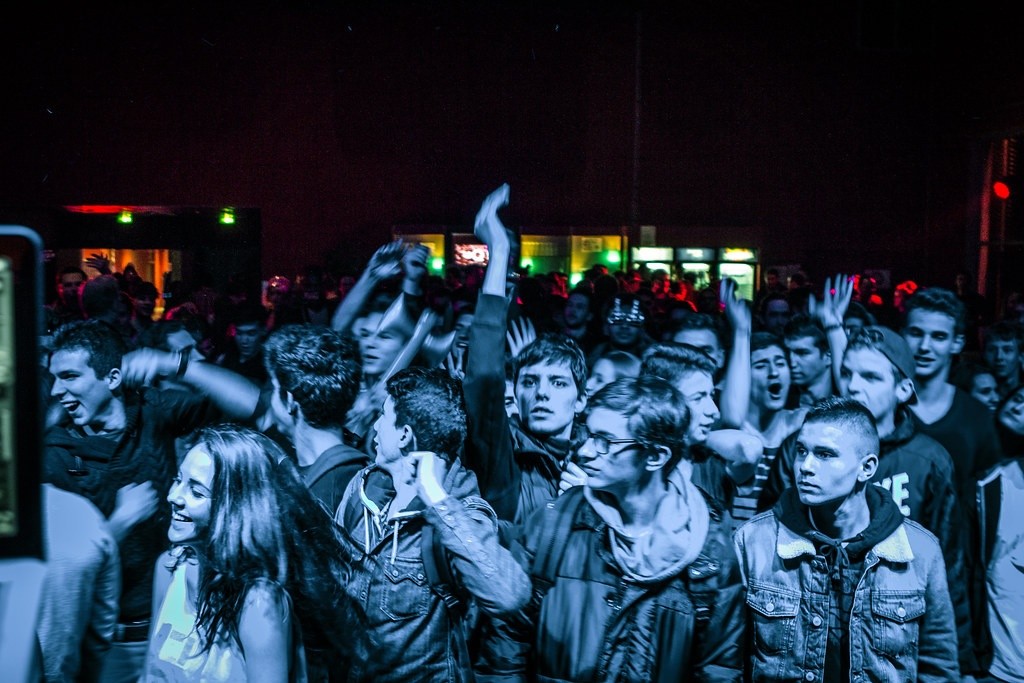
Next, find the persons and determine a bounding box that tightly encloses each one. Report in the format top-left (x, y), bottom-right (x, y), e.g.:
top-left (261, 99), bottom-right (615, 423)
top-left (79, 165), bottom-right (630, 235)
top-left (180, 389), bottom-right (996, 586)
top-left (0, 175), bottom-right (1024, 683)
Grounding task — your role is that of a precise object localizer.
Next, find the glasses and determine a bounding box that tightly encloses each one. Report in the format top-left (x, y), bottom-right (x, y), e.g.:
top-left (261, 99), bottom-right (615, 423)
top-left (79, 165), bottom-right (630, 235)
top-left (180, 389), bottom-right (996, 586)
top-left (606, 311), bottom-right (644, 326)
top-left (580, 423), bottom-right (642, 454)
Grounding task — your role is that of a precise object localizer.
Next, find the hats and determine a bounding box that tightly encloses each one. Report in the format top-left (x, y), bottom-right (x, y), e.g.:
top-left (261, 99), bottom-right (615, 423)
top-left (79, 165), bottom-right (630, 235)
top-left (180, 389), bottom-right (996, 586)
top-left (848, 324), bottom-right (920, 406)
